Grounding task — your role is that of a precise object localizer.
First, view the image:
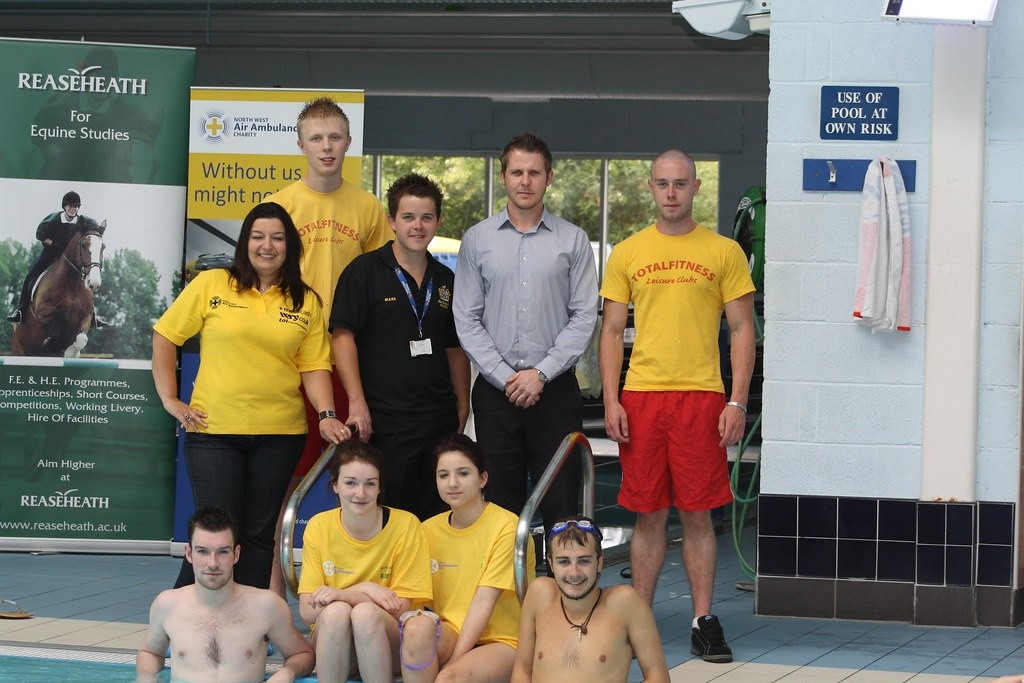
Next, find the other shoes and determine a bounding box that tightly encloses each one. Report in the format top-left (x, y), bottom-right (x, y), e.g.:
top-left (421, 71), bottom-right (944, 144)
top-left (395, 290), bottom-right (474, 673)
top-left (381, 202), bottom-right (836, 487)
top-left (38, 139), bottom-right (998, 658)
top-left (6, 307), bottom-right (23, 322)
top-left (91, 319), bottom-right (108, 327)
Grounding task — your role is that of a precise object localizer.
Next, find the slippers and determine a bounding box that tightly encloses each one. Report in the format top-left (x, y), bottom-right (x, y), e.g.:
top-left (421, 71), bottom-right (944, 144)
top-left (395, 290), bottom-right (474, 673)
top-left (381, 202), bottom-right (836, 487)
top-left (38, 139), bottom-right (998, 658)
top-left (0, 598), bottom-right (33, 618)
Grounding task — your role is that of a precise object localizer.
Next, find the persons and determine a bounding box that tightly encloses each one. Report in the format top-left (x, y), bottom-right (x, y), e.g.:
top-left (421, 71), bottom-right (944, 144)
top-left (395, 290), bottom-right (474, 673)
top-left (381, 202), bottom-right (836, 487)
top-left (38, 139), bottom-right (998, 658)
top-left (452, 134), bottom-right (598, 578)
top-left (7, 191), bottom-right (113, 328)
top-left (328, 173), bottom-right (471, 522)
top-left (598, 150), bottom-right (756, 663)
top-left (151, 202), bottom-right (352, 590)
top-left (136, 506), bottom-right (315, 683)
top-left (297, 439), bottom-right (433, 683)
top-left (261, 96), bottom-right (397, 602)
top-left (510, 516), bottom-right (671, 683)
top-left (399, 432), bottom-right (536, 683)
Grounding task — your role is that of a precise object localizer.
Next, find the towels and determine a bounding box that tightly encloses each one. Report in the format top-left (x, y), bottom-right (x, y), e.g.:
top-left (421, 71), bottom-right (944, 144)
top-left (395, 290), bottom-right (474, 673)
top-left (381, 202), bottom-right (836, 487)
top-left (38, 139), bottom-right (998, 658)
top-left (850, 155), bottom-right (914, 336)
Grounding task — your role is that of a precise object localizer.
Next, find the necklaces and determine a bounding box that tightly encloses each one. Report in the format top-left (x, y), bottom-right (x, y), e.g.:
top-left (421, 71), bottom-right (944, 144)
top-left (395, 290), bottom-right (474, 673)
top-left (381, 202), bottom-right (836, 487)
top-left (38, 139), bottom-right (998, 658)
top-left (561, 587), bottom-right (602, 640)
top-left (259, 286), bottom-right (268, 292)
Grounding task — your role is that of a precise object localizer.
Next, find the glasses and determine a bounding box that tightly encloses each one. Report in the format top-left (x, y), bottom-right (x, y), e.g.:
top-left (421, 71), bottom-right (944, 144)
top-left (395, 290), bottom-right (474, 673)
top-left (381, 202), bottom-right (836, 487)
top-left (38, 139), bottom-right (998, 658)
top-left (548, 520), bottom-right (601, 543)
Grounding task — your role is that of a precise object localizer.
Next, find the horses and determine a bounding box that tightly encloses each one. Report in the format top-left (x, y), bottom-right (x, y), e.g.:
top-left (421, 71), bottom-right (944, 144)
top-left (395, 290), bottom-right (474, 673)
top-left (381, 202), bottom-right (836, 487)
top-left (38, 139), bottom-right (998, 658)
top-left (11, 213), bottom-right (108, 359)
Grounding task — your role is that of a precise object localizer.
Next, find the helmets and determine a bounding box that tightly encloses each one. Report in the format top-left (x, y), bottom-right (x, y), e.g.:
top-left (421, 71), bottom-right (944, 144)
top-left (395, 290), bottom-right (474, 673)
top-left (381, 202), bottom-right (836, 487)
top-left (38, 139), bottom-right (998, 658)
top-left (62, 191), bottom-right (81, 208)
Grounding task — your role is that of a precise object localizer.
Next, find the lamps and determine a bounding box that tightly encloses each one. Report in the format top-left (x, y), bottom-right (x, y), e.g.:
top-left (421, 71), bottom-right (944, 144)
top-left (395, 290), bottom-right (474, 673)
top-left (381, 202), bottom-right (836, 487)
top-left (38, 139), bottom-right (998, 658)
top-left (671, 0), bottom-right (770, 41)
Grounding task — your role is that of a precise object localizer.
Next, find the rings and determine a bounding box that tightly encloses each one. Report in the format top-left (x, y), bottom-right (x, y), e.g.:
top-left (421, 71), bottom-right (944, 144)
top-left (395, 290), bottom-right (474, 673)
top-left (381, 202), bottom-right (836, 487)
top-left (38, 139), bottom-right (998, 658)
top-left (341, 429), bottom-right (346, 432)
top-left (185, 414), bottom-right (191, 422)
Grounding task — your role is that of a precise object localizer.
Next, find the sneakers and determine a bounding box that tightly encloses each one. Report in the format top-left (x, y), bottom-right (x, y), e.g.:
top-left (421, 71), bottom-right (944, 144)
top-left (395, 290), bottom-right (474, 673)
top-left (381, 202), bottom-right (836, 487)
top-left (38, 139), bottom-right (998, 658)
top-left (690, 614), bottom-right (733, 662)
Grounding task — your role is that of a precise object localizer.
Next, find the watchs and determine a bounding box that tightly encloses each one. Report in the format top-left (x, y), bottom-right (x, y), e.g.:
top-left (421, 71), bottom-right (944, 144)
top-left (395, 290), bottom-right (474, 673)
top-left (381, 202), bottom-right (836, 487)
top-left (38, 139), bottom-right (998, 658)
top-left (537, 369), bottom-right (549, 384)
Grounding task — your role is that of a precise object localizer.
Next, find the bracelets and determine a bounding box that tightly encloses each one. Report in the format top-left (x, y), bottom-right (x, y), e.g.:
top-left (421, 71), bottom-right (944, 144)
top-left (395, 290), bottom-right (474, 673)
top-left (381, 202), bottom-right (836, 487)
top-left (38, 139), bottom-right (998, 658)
top-left (724, 401), bottom-right (747, 413)
top-left (319, 410), bottom-right (336, 420)
